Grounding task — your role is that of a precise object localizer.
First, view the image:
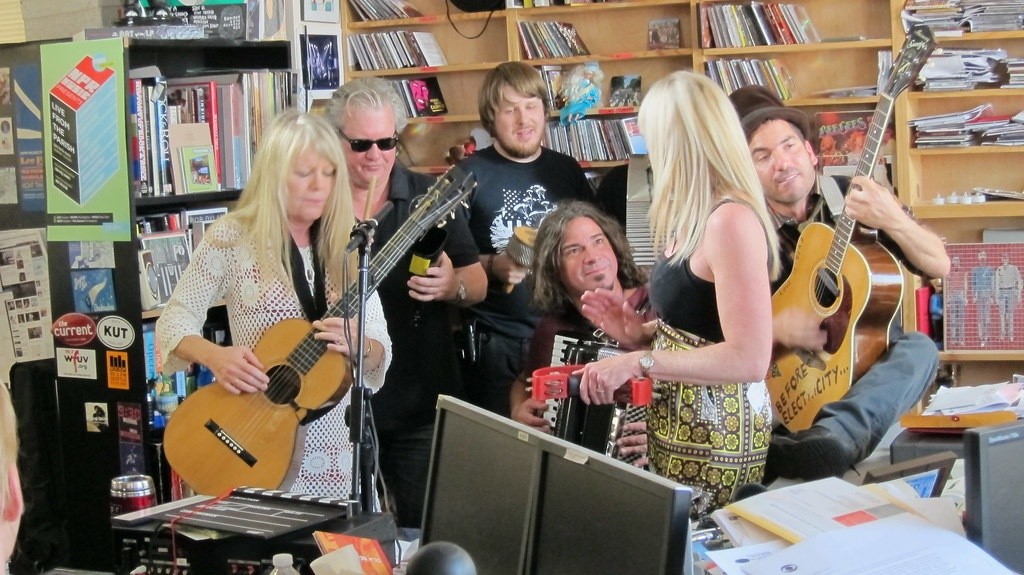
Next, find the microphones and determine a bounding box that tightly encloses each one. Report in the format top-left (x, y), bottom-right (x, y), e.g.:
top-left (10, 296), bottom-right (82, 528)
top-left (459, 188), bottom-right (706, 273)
top-left (346, 201), bottom-right (394, 253)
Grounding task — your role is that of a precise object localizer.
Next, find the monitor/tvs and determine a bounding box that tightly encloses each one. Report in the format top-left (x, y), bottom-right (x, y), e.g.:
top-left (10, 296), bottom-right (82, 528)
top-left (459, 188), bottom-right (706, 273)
top-left (414, 393), bottom-right (693, 575)
top-left (963, 420), bottom-right (1024, 575)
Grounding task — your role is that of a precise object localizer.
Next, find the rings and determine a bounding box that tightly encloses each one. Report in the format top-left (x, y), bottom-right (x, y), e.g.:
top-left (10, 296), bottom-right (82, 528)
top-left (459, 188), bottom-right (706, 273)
top-left (338, 335), bottom-right (341, 343)
top-left (596, 390), bottom-right (604, 393)
top-left (234, 380), bottom-right (238, 386)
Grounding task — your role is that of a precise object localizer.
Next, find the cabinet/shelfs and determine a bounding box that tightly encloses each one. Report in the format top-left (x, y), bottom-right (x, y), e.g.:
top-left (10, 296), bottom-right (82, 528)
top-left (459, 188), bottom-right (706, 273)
top-left (0, 0), bottom-right (1024, 533)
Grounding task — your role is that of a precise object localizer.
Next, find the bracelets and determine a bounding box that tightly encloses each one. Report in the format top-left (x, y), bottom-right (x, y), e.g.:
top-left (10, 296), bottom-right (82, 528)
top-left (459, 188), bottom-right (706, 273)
top-left (365, 340), bottom-right (372, 358)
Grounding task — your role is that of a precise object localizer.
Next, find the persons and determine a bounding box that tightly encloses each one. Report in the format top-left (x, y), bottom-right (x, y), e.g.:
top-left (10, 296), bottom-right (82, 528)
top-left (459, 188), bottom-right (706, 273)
top-left (328, 78), bottom-right (488, 528)
top-left (559, 61), bottom-right (604, 126)
top-left (929, 277), bottom-right (943, 342)
top-left (0, 383), bottom-right (23, 575)
top-left (819, 127), bottom-right (896, 164)
top-left (447, 61), bottom-right (594, 394)
top-left (511, 203), bottom-right (649, 468)
top-left (728, 85), bottom-right (951, 480)
top-left (572, 72), bottom-right (781, 529)
top-left (156, 112), bottom-right (392, 506)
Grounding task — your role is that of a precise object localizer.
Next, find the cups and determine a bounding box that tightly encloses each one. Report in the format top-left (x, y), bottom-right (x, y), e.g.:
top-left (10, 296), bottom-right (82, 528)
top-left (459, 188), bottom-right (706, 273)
top-left (155, 394), bottom-right (179, 418)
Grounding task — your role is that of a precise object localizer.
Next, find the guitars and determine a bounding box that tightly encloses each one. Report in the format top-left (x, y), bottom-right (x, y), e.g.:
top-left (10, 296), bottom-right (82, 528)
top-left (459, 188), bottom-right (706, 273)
top-left (163, 164), bottom-right (477, 496)
top-left (765, 25), bottom-right (941, 433)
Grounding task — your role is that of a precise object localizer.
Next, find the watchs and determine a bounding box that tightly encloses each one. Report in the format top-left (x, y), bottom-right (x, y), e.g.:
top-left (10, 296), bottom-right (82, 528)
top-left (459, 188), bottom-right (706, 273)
top-left (454, 280), bottom-right (466, 304)
top-left (639, 349), bottom-right (654, 378)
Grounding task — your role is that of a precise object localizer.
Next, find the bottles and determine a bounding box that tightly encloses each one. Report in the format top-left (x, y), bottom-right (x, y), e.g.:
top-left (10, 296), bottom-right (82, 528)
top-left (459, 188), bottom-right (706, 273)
top-left (147, 393), bottom-right (154, 425)
top-left (269, 553), bottom-right (300, 575)
top-left (197, 364), bottom-right (216, 389)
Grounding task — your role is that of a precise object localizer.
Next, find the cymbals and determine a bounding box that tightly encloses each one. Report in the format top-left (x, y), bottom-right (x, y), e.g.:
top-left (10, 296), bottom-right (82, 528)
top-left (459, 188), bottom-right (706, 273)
top-left (524, 363), bottom-right (654, 411)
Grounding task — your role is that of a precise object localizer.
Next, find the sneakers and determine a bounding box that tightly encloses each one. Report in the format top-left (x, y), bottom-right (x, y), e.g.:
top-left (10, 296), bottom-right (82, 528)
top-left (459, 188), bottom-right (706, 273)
top-left (764, 424), bottom-right (846, 481)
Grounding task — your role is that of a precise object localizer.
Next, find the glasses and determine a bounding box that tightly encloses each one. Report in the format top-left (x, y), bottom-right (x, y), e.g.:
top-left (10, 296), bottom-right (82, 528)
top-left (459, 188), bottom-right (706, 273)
top-left (339, 129), bottom-right (399, 153)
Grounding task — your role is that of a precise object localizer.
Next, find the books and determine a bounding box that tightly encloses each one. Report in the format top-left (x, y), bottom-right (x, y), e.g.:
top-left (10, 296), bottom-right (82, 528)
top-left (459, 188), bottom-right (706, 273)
top-left (899, 0), bottom-right (1024, 349)
top-left (313, 532), bottom-right (393, 575)
top-left (712, 477), bottom-right (919, 546)
top-left (129, 70), bottom-right (298, 395)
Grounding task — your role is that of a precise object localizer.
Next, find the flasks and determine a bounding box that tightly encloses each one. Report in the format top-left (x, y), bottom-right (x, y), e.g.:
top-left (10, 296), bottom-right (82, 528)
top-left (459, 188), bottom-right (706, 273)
top-left (110, 475), bottom-right (157, 518)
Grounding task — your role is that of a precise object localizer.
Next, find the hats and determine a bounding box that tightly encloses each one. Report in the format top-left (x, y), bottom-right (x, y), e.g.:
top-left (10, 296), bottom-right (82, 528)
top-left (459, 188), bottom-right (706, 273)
top-left (726, 85), bottom-right (811, 140)
